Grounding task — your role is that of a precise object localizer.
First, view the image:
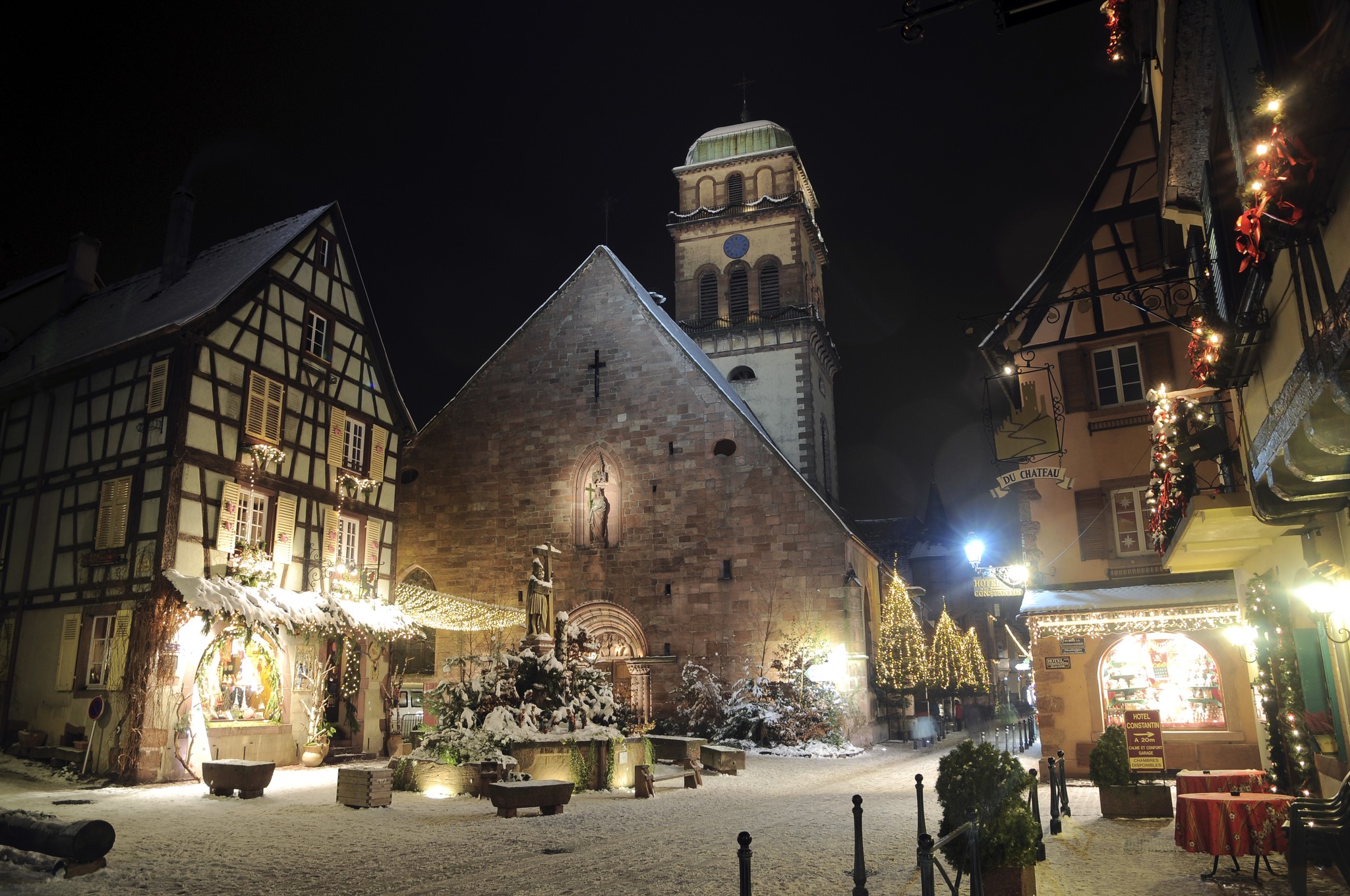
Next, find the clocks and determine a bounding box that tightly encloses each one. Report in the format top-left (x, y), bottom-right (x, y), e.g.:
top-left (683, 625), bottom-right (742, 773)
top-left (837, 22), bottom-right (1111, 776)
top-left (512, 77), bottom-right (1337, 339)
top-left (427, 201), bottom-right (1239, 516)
top-left (723, 234), bottom-right (749, 259)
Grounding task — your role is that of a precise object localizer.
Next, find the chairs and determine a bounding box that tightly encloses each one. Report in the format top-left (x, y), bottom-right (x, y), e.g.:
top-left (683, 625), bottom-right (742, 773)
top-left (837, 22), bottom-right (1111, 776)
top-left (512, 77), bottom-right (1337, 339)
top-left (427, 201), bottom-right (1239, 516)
top-left (1280, 771), bottom-right (1350, 896)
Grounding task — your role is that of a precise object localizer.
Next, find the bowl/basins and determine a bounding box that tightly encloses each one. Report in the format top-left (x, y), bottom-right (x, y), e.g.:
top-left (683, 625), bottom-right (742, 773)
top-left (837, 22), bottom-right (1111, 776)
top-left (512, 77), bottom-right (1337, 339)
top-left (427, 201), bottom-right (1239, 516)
top-left (1203, 771), bottom-right (1209, 774)
top-left (1130, 697), bottom-right (1135, 700)
top-left (1230, 791), bottom-right (1241, 796)
top-left (1115, 697), bottom-right (1125, 701)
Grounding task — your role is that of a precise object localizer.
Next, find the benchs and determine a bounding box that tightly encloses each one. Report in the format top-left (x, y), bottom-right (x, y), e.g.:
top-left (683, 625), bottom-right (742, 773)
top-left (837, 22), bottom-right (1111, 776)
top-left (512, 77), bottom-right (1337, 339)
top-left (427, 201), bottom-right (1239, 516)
top-left (488, 779), bottom-right (575, 819)
top-left (202, 758), bottom-right (276, 800)
top-left (634, 734), bottom-right (746, 800)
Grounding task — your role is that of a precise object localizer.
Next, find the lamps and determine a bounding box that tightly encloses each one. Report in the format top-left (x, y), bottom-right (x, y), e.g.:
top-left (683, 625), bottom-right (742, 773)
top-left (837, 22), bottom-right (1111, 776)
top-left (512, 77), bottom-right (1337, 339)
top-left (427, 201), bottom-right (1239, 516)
top-left (964, 532), bottom-right (1024, 591)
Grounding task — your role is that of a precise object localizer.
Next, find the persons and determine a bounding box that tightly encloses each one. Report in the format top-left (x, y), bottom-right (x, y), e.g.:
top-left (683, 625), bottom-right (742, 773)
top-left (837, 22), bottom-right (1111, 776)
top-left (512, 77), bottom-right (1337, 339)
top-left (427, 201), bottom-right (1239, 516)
top-left (930, 699), bottom-right (944, 732)
top-left (953, 700), bottom-right (962, 732)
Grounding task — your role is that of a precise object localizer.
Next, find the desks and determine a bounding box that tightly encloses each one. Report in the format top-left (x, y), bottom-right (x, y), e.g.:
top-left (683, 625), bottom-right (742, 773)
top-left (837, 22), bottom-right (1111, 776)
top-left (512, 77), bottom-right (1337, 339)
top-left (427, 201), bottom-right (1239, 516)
top-left (1175, 769), bottom-right (1296, 885)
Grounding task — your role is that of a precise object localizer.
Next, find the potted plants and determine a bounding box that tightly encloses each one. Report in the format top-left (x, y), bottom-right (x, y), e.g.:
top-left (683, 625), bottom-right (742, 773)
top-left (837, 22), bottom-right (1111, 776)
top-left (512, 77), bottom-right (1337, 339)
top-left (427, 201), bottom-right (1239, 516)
top-left (934, 740), bottom-right (1041, 896)
top-left (1090, 724), bottom-right (1174, 819)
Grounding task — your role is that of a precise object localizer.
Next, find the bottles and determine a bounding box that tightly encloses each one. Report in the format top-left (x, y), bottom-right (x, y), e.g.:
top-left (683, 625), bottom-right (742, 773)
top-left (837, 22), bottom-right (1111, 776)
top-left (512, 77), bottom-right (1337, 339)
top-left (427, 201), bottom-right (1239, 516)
top-left (1106, 705), bottom-right (1123, 725)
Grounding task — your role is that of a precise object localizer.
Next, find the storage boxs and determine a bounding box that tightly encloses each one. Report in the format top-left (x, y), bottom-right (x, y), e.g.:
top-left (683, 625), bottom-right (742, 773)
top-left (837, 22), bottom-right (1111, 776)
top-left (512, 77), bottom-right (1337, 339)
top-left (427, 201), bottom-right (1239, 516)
top-left (335, 768), bottom-right (394, 807)
